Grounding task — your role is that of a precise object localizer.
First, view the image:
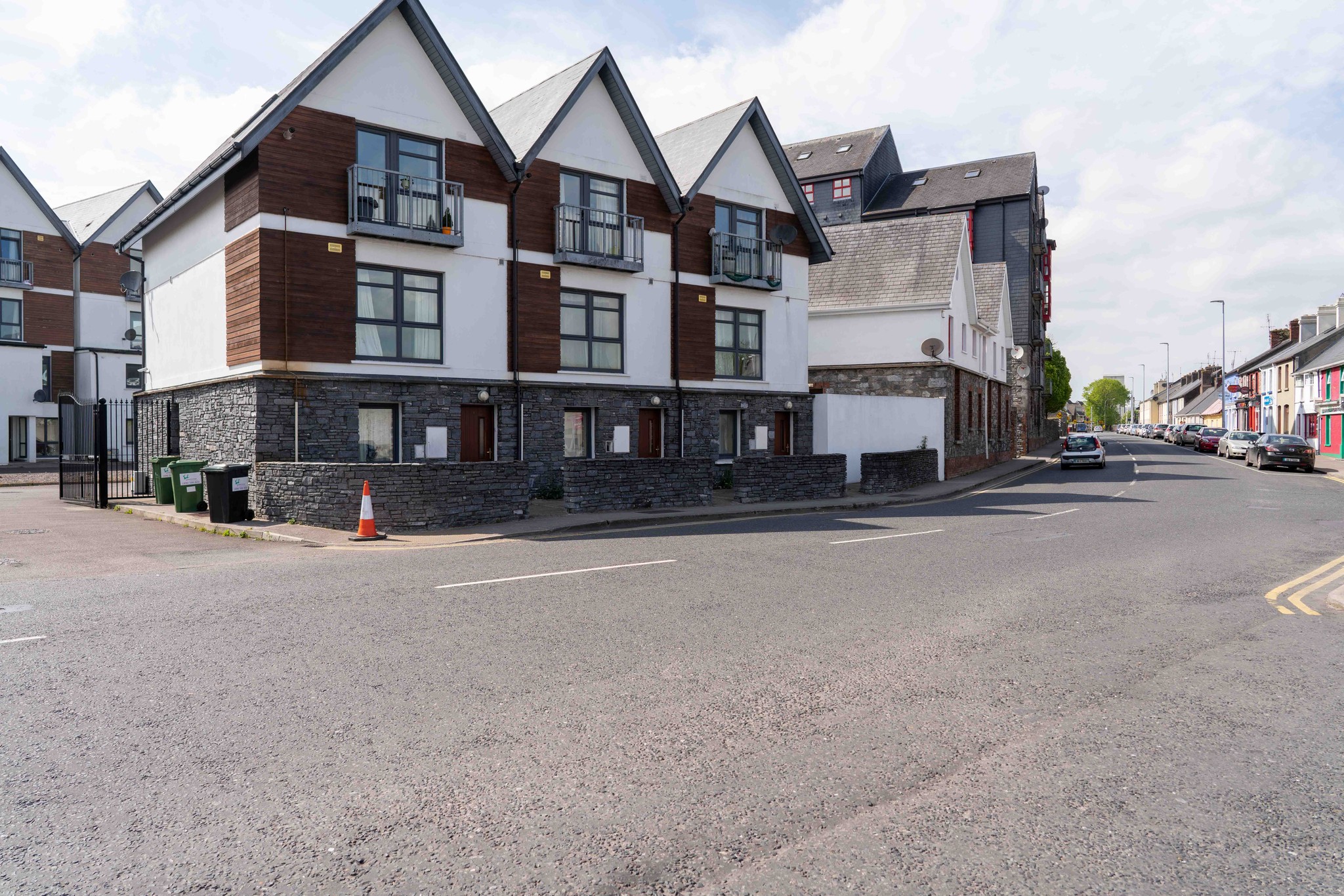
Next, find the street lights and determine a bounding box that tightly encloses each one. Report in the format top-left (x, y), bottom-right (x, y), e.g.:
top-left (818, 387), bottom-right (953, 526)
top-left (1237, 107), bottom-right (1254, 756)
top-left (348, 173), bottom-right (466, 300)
top-left (1210, 300), bottom-right (1225, 427)
top-left (1159, 342), bottom-right (1169, 424)
top-left (1128, 377), bottom-right (1133, 424)
top-left (1104, 398), bottom-right (1115, 431)
top-left (1138, 364), bottom-right (1144, 424)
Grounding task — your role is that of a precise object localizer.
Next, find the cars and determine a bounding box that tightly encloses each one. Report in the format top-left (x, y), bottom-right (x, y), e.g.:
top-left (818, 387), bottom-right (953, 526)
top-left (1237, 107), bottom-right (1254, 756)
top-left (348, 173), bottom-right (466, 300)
top-left (1094, 426), bottom-right (1103, 433)
top-left (1060, 433), bottom-right (1108, 469)
top-left (1245, 434), bottom-right (1316, 473)
top-left (1168, 424), bottom-right (1183, 444)
top-left (1217, 430), bottom-right (1262, 460)
top-left (1115, 424), bottom-right (1154, 438)
top-left (1162, 424), bottom-right (1177, 443)
top-left (1194, 427), bottom-right (1230, 455)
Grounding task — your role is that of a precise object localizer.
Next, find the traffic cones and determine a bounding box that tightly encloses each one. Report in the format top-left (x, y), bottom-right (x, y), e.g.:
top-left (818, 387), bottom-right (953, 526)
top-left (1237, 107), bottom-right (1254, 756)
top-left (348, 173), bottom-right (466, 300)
top-left (347, 479), bottom-right (388, 541)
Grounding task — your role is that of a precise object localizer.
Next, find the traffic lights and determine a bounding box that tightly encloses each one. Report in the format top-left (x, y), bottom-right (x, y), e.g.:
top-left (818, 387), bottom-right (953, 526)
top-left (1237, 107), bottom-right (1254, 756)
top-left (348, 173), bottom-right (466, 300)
top-left (1083, 416), bottom-right (1086, 421)
top-left (1086, 417), bottom-right (1089, 421)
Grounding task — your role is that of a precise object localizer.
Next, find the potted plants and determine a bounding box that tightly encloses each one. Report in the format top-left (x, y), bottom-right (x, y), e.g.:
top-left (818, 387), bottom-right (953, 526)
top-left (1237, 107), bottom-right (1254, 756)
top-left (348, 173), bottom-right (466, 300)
top-left (442, 207), bottom-right (453, 234)
top-left (400, 176), bottom-right (414, 189)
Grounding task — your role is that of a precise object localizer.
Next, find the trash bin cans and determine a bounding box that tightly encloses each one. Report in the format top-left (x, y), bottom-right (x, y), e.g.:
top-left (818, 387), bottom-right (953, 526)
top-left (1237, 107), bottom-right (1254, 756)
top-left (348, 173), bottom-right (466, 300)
top-left (199, 464), bottom-right (255, 524)
top-left (148, 455), bottom-right (182, 504)
top-left (167, 460), bottom-right (208, 513)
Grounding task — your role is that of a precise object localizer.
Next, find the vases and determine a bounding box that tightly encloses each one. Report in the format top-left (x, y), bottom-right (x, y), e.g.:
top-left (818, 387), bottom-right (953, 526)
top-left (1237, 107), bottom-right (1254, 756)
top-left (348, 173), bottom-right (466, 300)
top-left (767, 276), bottom-right (775, 281)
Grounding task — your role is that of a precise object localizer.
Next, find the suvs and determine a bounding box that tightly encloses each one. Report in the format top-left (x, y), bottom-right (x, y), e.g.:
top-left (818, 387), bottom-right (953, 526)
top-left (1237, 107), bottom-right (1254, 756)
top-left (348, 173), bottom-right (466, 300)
top-left (1175, 423), bottom-right (1210, 447)
top-left (1150, 423), bottom-right (1170, 440)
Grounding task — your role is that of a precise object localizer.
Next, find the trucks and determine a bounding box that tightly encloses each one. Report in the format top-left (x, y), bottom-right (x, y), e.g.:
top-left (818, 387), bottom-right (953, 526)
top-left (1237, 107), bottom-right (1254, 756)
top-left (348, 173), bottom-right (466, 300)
top-left (1076, 423), bottom-right (1087, 433)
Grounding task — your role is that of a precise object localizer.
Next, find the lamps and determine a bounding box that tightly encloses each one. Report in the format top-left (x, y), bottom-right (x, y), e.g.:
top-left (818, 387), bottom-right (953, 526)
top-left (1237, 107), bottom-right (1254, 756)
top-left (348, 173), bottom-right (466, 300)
top-left (785, 401), bottom-right (792, 409)
top-left (651, 396), bottom-right (660, 406)
top-left (478, 391), bottom-right (489, 403)
